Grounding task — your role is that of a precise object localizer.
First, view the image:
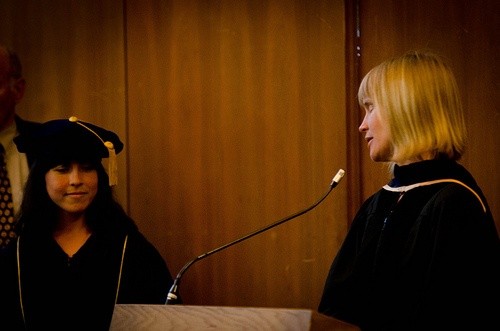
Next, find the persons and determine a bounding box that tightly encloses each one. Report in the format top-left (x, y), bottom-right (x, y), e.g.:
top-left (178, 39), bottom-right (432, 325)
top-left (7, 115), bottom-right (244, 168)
top-left (0, 47), bottom-right (41, 252)
top-left (0, 117), bottom-right (178, 331)
top-left (317, 50), bottom-right (500, 331)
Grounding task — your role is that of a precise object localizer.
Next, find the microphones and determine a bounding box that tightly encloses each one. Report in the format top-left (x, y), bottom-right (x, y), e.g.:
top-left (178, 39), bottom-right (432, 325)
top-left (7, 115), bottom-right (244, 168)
top-left (167, 168), bottom-right (346, 305)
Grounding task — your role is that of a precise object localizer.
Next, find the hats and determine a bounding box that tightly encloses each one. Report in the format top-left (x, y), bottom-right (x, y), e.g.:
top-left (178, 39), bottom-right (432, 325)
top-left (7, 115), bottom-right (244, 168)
top-left (13, 113), bottom-right (124, 186)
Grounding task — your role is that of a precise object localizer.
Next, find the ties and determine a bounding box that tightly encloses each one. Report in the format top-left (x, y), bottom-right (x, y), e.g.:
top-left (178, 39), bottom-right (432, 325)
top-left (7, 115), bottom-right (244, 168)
top-left (0, 144), bottom-right (16, 248)
